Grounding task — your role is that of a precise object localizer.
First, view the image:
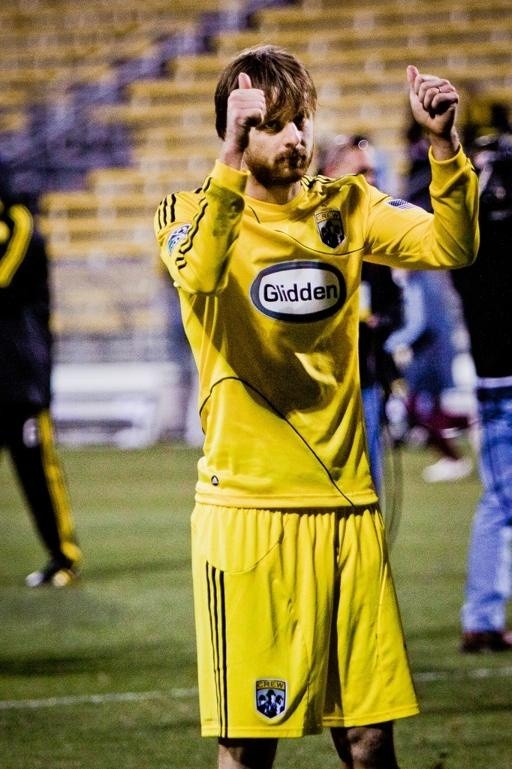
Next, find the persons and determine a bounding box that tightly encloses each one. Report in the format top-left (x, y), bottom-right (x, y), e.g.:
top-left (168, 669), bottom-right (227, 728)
top-left (150, 258), bottom-right (202, 451)
top-left (448, 100), bottom-right (512, 657)
top-left (1, 154), bottom-right (88, 594)
top-left (152, 42), bottom-right (484, 769)
top-left (319, 120), bottom-right (474, 481)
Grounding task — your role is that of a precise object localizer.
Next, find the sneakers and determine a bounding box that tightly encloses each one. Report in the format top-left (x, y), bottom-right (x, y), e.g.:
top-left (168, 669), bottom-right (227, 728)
top-left (422, 458), bottom-right (473, 482)
top-left (25, 560), bottom-right (79, 589)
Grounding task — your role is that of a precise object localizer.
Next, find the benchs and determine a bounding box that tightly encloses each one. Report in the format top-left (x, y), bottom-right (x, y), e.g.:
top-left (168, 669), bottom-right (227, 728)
top-left (1, 0), bottom-right (512, 442)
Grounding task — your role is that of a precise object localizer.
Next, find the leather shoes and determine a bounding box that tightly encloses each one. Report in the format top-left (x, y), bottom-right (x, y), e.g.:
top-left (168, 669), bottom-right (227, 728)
top-left (460, 630), bottom-right (512, 653)
